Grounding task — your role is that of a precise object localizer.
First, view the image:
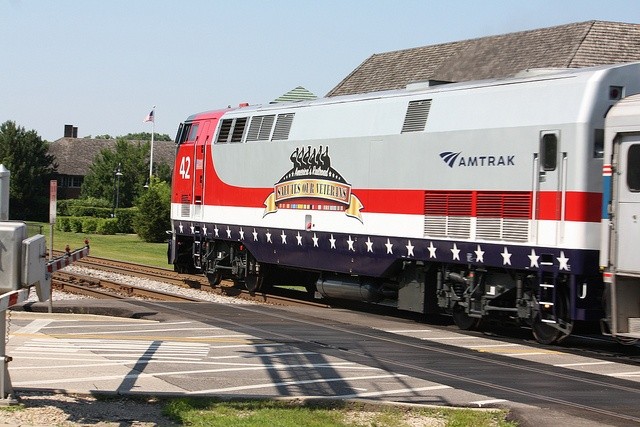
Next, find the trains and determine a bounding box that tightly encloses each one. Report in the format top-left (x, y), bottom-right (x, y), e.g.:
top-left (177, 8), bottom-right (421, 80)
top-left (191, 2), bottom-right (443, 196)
top-left (166, 60), bottom-right (640, 346)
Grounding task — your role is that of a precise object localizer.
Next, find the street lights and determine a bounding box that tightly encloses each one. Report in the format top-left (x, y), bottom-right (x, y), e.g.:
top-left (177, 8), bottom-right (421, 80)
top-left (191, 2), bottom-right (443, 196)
top-left (114, 163), bottom-right (123, 218)
